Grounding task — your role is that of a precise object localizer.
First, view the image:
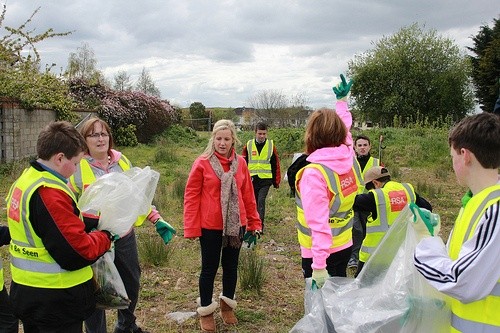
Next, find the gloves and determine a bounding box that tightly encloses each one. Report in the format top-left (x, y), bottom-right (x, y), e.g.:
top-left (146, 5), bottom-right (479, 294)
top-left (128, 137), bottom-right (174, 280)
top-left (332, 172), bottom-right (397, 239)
top-left (332, 73), bottom-right (352, 99)
top-left (310, 267), bottom-right (329, 288)
top-left (155, 218), bottom-right (176, 244)
top-left (410, 202), bottom-right (444, 241)
top-left (243, 231), bottom-right (258, 248)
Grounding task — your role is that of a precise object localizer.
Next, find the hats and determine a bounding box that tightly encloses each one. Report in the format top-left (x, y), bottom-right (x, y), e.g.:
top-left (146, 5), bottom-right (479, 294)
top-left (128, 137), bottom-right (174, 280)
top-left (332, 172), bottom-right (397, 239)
top-left (363, 166), bottom-right (391, 186)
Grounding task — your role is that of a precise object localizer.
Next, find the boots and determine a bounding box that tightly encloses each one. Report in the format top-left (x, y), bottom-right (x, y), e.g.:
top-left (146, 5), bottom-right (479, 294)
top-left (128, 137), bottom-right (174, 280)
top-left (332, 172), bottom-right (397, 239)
top-left (220, 292), bottom-right (239, 327)
top-left (195, 296), bottom-right (217, 333)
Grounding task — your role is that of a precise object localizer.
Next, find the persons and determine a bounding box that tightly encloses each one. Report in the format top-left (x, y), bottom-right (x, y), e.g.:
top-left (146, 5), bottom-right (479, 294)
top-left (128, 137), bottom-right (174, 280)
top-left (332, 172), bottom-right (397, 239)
top-left (7, 119), bottom-right (120, 333)
top-left (408, 112), bottom-right (500, 333)
top-left (288, 73), bottom-right (369, 290)
top-left (183, 119), bottom-right (262, 332)
top-left (240, 122), bottom-right (281, 235)
top-left (64, 117), bottom-right (177, 332)
top-left (354, 165), bottom-right (432, 281)
top-left (354, 134), bottom-right (384, 176)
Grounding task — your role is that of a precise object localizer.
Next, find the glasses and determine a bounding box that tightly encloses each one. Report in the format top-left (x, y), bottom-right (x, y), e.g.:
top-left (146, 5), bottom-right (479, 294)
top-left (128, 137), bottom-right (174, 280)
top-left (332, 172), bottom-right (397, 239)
top-left (85, 132), bottom-right (110, 138)
